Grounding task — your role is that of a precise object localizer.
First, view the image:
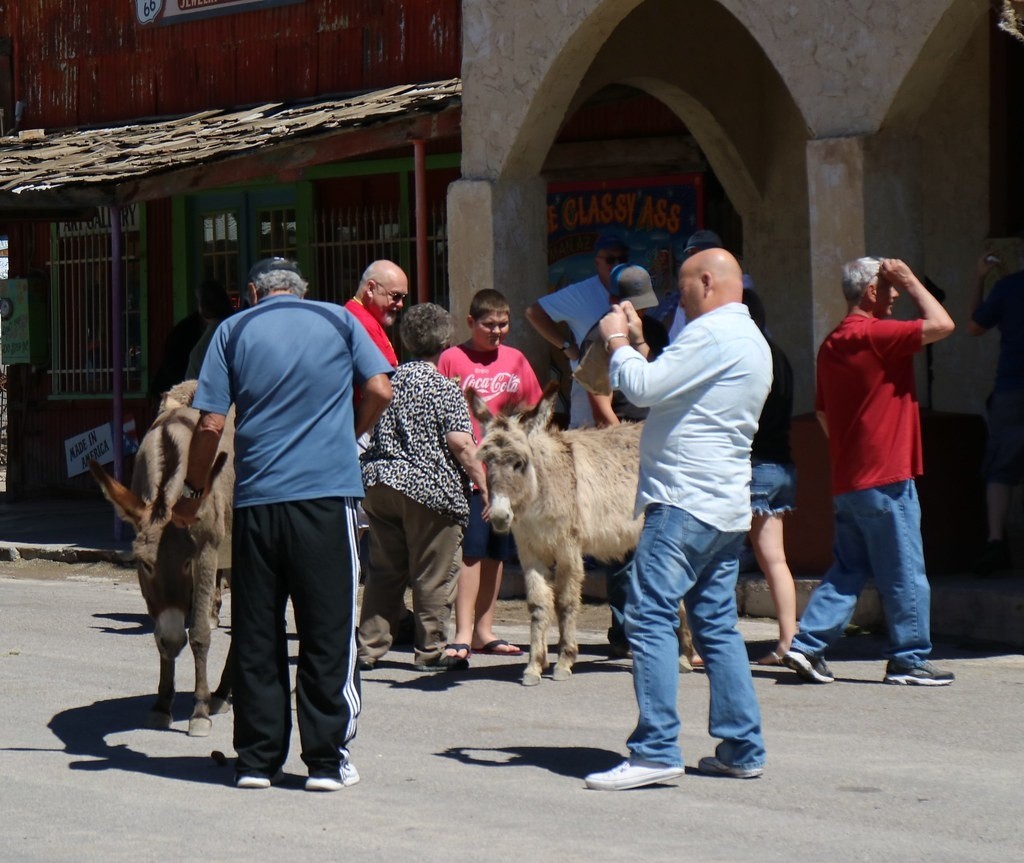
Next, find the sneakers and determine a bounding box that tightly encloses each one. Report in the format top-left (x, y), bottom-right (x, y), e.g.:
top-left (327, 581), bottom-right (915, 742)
top-left (413, 654), bottom-right (470, 672)
top-left (585, 753), bottom-right (685, 792)
top-left (233, 767), bottom-right (284, 787)
top-left (883, 659), bottom-right (955, 686)
top-left (360, 660), bottom-right (374, 670)
top-left (305, 764), bottom-right (360, 790)
top-left (782, 649), bottom-right (835, 683)
top-left (698, 757), bottom-right (763, 779)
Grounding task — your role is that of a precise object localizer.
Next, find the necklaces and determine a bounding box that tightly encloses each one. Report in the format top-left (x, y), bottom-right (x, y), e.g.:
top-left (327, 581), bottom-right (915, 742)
top-left (353, 296), bottom-right (368, 309)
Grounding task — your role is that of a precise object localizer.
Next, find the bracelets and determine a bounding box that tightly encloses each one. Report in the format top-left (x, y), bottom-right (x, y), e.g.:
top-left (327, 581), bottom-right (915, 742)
top-left (632, 340), bottom-right (647, 347)
top-left (602, 332), bottom-right (630, 352)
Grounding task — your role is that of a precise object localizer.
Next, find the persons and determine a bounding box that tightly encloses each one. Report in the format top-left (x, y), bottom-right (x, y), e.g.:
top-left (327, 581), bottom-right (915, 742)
top-left (782, 255), bottom-right (957, 689)
top-left (348, 260), bottom-right (420, 649)
top-left (169, 256), bottom-right (394, 793)
top-left (185, 280), bottom-right (237, 386)
top-left (584, 248), bottom-right (763, 793)
top-left (438, 290), bottom-right (558, 674)
top-left (964, 251), bottom-right (1024, 578)
top-left (355, 302), bottom-right (471, 672)
top-left (522, 230), bottom-right (799, 666)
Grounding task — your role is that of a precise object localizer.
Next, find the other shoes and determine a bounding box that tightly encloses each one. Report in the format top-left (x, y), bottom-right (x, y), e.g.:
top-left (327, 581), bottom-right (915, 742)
top-left (982, 539), bottom-right (1007, 564)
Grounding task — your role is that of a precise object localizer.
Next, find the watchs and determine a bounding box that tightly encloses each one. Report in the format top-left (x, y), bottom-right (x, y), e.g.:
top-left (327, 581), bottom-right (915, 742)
top-left (559, 339), bottom-right (572, 353)
top-left (182, 480), bottom-right (204, 501)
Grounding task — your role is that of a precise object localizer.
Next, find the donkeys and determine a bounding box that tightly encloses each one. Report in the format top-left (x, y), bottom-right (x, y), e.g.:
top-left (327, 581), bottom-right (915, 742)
top-left (86, 374), bottom-right (244, 737)
top-left (463, 385), bottom-right (694, 687)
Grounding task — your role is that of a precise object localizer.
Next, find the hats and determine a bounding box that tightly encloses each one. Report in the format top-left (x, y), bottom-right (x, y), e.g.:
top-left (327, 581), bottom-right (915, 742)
top-left (248, 256), bottom-right (306, 282)
top-left (594, 233), bottom-right (631, 256)
top-left (682, 229), bottom-right (724, 253)
top-left (609, 262), bottom-right (659, 311)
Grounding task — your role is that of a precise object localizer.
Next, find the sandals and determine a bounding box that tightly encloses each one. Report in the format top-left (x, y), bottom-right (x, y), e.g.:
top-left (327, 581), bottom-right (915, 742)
top-left (758, 650), bottom-right (789, 666)
top-left (692, 655), bottom-right (705, 669)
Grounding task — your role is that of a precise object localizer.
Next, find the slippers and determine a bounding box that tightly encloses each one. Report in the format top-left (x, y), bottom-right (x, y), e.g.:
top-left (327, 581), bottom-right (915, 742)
top-left (471, 640), bottom-right (524, 656)
top-left (444, 643), bottom-right (472, 661)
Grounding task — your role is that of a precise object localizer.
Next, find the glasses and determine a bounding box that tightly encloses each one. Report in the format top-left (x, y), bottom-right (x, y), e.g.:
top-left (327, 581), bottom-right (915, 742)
top-left (598, 255), bottom-right (629, 265)
top-left (375, 281), bottom-right (408, 302)
